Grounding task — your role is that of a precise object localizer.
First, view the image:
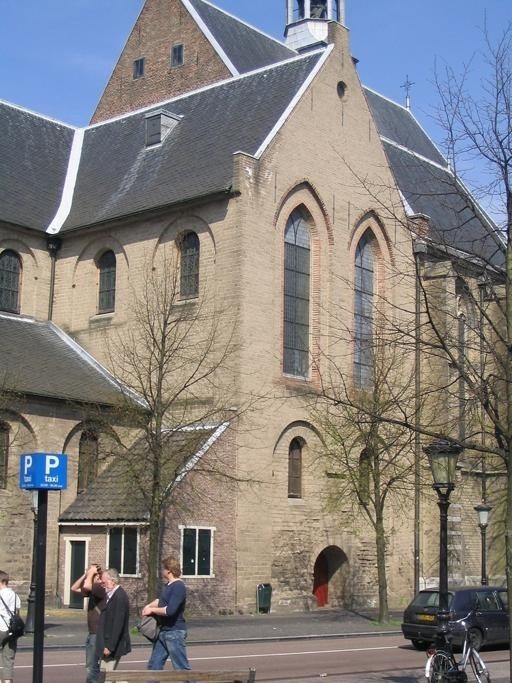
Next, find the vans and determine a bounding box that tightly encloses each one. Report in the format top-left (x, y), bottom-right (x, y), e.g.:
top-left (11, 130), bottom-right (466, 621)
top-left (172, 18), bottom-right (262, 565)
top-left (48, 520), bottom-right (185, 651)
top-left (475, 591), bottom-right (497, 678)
top-left (397, 582), bottom-right (512, 652)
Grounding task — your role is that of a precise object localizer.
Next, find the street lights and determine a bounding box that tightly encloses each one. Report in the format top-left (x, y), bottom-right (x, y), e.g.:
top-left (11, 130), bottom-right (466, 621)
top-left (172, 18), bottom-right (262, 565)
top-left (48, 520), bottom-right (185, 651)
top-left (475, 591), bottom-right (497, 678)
top-left (473, 497), bottom-right (493, 587)
top-left (421, 423), bottom-right (466, 681)
top-left (22, 489), bottom-right (39, 632)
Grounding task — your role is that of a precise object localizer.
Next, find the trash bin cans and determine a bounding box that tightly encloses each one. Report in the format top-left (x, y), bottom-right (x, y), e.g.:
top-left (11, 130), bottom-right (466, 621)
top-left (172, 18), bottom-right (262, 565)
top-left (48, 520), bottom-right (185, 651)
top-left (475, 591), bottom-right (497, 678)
top-left (257, 584), bottom-right (272, 616)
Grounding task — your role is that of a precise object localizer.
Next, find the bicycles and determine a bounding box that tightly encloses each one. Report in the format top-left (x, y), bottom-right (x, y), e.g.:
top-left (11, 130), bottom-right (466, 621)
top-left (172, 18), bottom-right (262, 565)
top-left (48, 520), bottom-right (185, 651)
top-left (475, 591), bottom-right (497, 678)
top-left (423, 606), bottom-right (493, 682)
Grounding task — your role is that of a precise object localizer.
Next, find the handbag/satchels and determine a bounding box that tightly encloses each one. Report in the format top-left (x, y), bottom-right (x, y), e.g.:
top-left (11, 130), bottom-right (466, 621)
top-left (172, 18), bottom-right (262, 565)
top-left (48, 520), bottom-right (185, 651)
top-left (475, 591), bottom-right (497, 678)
top-left (137, 616), bottom-right (163, 642)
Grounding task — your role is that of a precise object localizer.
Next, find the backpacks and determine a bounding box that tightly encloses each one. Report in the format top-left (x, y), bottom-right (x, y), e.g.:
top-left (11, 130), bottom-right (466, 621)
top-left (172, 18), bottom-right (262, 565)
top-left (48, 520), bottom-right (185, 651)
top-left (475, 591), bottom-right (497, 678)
top-left (0, 592), bottom-right (24, 640)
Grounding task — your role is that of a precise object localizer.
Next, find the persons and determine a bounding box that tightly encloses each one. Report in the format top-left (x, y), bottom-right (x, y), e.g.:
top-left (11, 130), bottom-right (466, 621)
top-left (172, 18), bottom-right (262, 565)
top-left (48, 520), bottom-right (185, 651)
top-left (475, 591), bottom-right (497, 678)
top-left (0, 570), bottom-right (22, 681)
top-left (95, 567), bottom-right (131, 671)
top-left (69, 562), bottom-right (107, 683)
top-left (142, 555), bottom-right (192, 669)
